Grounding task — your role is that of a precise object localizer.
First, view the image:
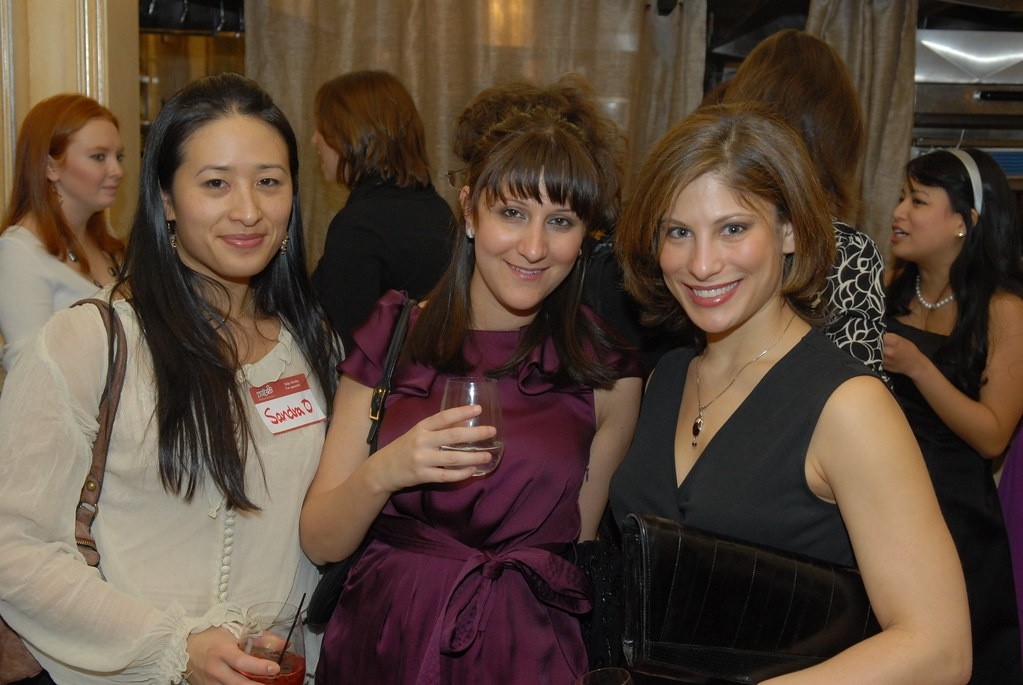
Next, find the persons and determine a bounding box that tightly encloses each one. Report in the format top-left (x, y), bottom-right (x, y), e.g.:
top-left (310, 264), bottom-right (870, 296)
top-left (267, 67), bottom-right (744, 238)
top-left (607, 100), bottom-right (974, 684)
top-left (309, 70), bottom-right (458, 362)
top-left (298, 72), bottom-right (643, 685)
top-left (578, 29), bottom-right (896, 395)
top-left (1, 71), bottom-right (346, 685)
top-left (882, 145), bottom-right (1023, 685)
top-left (1, 92), bottom-right (129, 382)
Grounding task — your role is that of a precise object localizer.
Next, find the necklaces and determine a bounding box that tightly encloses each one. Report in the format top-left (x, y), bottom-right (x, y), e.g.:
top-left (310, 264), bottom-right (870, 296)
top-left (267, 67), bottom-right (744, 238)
top-left (692, 313), bottom-right (796, 447)
top-left (221, 296), bottom-right (255, 321)
top-left (916, 275), bottom-right (954, 309)
top-left (67, 252), bottom-right (116, 288)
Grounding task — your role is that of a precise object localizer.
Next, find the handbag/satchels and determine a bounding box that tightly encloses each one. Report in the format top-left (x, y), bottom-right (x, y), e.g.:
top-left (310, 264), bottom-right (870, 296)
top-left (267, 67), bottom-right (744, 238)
top-left (0, 298), bottom-right (127, 685)
top-left (308, 298), bottom-right (417, 627)
top-left (617, 512), bottom-right (871, 683)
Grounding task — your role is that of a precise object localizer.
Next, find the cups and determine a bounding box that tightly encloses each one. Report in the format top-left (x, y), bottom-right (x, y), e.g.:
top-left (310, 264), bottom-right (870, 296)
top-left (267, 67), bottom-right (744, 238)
top-left (234, 601), bottom-right (306, 684)
top-left (437, 376), bottom-right (505, 477)
top-left (578, 667), bottom-right (633, 685)
